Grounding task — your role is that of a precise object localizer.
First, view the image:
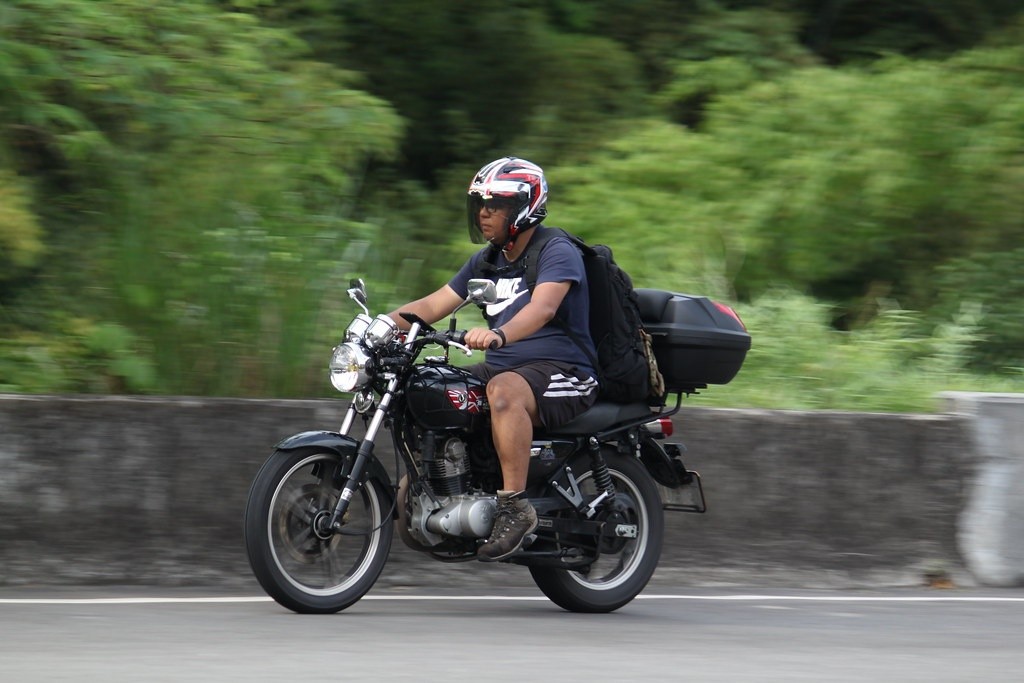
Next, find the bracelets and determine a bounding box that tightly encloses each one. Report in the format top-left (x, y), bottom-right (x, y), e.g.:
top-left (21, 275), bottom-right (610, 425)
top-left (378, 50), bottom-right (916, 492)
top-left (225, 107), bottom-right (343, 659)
top-left (491, 328), bottom-right (506, 349)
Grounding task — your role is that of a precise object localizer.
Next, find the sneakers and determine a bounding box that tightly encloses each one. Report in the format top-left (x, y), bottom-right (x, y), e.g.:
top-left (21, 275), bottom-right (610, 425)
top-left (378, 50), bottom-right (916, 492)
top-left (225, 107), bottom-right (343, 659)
top-left (477, 490), bottom-right (539, 563)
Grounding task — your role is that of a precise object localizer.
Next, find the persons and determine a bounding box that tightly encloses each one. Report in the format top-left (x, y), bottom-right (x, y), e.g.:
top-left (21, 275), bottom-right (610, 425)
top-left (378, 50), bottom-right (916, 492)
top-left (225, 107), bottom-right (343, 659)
top-left (385, 156), bottom-right (599, 563)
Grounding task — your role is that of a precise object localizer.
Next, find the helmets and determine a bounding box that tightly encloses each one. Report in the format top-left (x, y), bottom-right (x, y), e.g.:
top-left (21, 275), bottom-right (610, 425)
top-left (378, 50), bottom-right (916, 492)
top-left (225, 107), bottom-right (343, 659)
top-left (467, 157), bottom-right (547, 237)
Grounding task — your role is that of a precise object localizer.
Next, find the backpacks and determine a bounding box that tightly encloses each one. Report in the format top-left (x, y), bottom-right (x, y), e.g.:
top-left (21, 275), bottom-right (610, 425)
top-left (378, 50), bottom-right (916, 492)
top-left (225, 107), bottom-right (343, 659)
top-left (485, 227), bottom-right (666, 406)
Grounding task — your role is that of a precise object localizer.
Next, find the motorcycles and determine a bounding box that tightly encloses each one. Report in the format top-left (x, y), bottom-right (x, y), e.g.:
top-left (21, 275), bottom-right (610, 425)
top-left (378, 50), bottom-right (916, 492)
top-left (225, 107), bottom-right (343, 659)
top-left (242, 276), bottom-right (751, 614)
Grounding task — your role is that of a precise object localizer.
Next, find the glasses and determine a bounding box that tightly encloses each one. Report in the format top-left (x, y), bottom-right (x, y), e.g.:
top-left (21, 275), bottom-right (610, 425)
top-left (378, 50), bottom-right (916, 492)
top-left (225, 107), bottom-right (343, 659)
top-left (479, 199), bottom-right (519, 213)
